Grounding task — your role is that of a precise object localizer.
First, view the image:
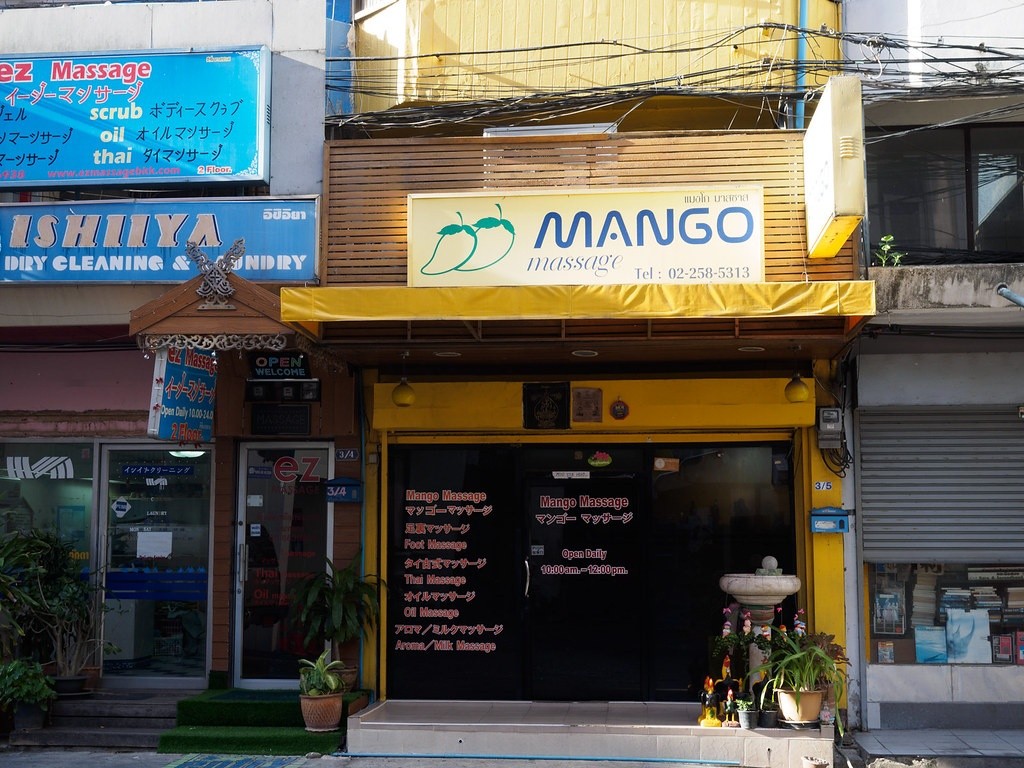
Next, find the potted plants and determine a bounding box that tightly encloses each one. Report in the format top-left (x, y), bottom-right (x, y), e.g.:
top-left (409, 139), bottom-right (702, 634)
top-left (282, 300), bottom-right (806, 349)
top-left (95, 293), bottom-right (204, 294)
top-left (298, 649), bottom-right (346, 732)
top-left (712, 620), bottom-right (851, 728)
top-left (291, 545), bottom-right (389, 689)
top-left (24, 558), bottom-right (130, 698)
